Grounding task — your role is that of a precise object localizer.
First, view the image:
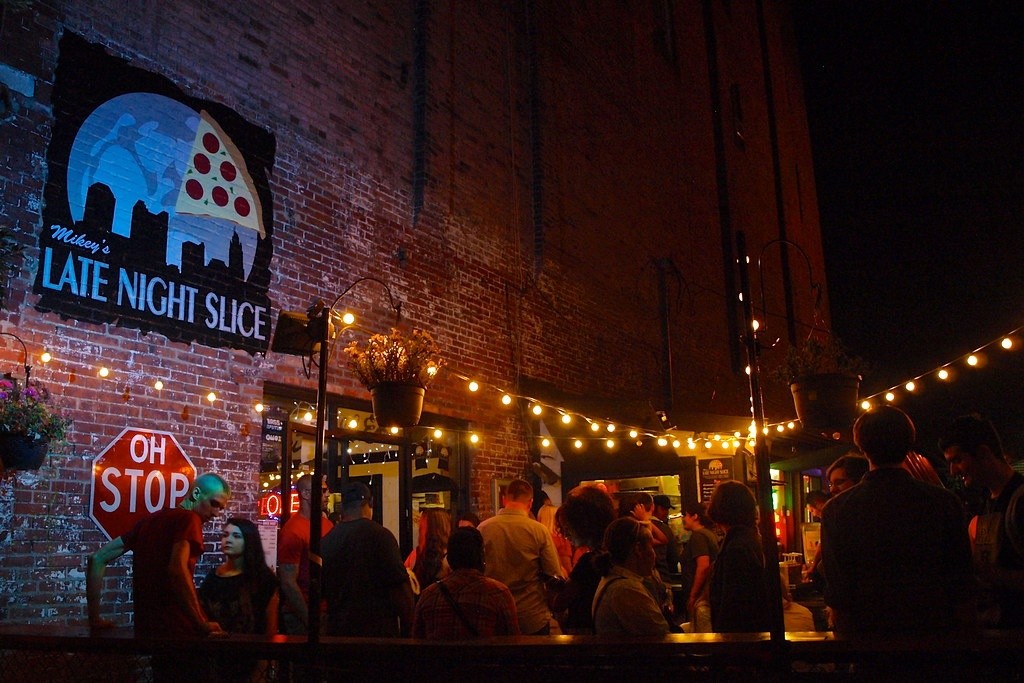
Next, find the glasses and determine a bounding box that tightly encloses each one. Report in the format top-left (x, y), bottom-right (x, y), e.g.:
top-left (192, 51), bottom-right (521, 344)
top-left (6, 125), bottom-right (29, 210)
top-left (307, 487), bottom-right (331, 493)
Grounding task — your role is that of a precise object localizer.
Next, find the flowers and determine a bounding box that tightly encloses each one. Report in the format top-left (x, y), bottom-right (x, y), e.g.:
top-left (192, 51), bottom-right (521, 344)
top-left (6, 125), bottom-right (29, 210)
top-left (0, 373), bottom-right (73, 443)
top-left (777, 332), bottom-right (861, 381)
top-left (344, 322), bottom-right (446, 388)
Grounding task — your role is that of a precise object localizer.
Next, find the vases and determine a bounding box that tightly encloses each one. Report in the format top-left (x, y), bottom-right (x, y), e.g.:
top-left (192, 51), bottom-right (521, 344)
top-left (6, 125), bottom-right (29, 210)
top-left (0, 431), bottom-right (51, 470)
top-left (369, 383), bottom-right (428, 428)
top-left (787, 373), bottom-right (862, 429)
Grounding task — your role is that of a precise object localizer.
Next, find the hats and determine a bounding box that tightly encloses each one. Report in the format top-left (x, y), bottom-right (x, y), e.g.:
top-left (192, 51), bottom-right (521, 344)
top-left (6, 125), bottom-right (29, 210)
top-left (341, 481), bottom-right (372, 503)
top-left (654, 495), bottom-right (675, 509)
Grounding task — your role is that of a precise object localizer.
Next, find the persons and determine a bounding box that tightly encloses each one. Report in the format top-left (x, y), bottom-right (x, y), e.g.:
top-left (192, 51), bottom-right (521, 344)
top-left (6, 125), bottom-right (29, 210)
top-left (277, 474), bottom-right (334, 636)
top-left (322, 484), bottom-right (415, 635)
top-left (807, 404), bottom-right (1024, 683)
top-left (404, 480), bottom-right (720, 634)
top-left (87, 474), bottom-right (222, 683)
top-left (199, 517), bottom-right (279, 683)
top-left (707, 479), bottom-right (772, 634)
top-left (413, 528), bottom-right (521, 637)
top-left (592, 518), bottom-right (699, 671)
